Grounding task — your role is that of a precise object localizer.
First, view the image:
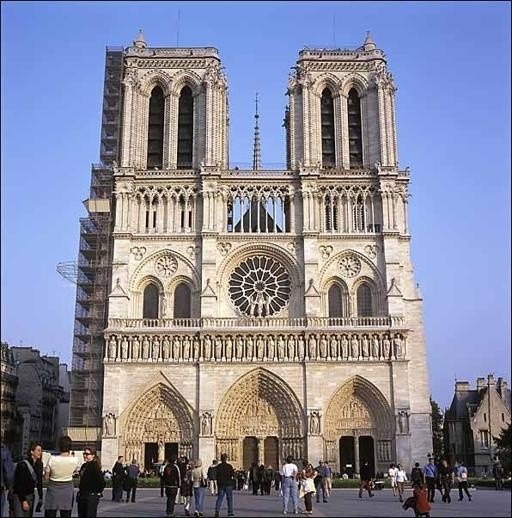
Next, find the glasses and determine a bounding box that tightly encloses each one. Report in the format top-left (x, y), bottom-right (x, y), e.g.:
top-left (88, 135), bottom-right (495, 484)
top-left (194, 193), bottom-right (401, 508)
top-left (83, 452), bottom-right (93, 455)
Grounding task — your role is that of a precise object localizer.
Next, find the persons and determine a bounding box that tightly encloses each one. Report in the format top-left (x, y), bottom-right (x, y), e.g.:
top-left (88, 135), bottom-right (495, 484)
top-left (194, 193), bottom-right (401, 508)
top-left (402, 452), bottom-right (472, 517)
top-left (160, 456), bottom-right (207, 516)
top-left (0, 435), bottom-right (106, 518)
top-left (360, 460), bottom-right (375, 498)
top-left (387, 464), bottom-right (411, 496)
top-left (493, 462), bottom-right (503, 490)
top-left (111, 456), bottom-right (141, 502)
top-left (208, 453), bottom-right (331, 516)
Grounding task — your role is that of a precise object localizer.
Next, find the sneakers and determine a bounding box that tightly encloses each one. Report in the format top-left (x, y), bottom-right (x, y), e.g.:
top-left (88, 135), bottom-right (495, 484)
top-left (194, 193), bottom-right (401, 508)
top-left (184, 507), bottom-right (202, 516)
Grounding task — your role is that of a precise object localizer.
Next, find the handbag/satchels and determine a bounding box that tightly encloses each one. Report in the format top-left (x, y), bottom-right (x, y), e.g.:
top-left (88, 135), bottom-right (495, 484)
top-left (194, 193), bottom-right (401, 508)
top-left (201, 478), bottom-right (208, 487)
